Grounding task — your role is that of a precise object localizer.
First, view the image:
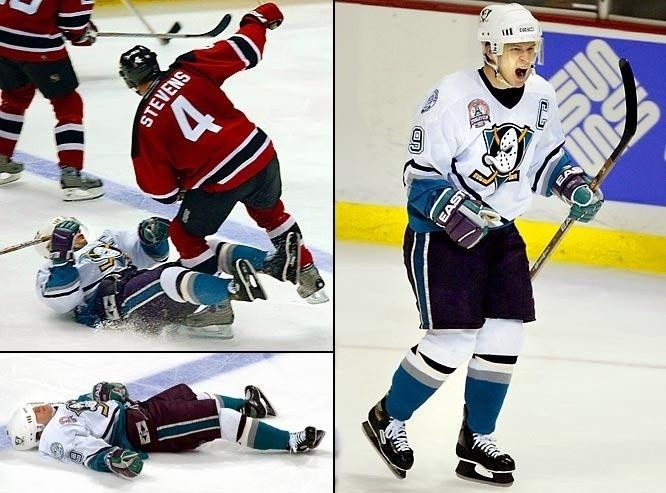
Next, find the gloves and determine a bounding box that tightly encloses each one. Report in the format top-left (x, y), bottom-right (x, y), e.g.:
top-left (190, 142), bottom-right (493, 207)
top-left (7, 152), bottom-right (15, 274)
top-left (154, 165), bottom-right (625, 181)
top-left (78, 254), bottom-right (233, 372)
top-left (92, 382), bottom-right (128, 403)
top-left (105, 446), bottom-right (142, 479)
top-left (138, 218), bottom-right (171, 247)
top-left (240, 3), bottom-right (283, 29)
top-left (430, 188), bottom-right (504, 250)
top-left (74, 22), bottom-right (98, 47)
top-left (551, 165), bottom-right (604, 222)
top-left (51, 221), bottom-right (78, 252)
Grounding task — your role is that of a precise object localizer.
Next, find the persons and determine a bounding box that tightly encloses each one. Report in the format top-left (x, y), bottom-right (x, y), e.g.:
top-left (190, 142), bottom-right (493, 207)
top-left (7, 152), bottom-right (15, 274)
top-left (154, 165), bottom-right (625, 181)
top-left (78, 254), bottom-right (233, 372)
top-left (0, 0), bottom-right (106, 202)
top-left (118, 1), bottom-right (329, 339)
top-left (34, 215), bottom-right (301, 334)
top-left (6, 379), bottom-right (326, 479)
top-left (361, 1), bottom-right (604, 487)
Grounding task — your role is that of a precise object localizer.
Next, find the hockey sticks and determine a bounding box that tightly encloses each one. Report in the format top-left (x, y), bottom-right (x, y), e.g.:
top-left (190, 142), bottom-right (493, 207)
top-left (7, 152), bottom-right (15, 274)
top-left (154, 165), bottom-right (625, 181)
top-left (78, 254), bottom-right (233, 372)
top-left (124, 0), bottom-right (180, 43)
top-left (96, 13), bottom-right (232, 37)
top-left (529, 57), bottom-right (638, 283)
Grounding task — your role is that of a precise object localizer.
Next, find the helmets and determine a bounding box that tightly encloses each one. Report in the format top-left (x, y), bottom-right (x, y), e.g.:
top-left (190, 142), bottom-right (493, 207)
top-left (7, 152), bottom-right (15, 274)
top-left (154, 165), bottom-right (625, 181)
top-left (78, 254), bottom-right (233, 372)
top-left (477, 4), bottom-right (543, 57)
top-left (5, 404), bottom-right (44, 451)
top-left (118, 46), bottom-right (159, 87)
top-left (37, 216), bottom-right (91, 259)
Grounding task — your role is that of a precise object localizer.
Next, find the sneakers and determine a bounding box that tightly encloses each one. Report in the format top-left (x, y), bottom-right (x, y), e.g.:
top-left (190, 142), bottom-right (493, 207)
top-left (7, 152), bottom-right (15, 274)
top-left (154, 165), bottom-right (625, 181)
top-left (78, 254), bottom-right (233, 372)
top-left (286, 427), bottom-right (317, 452)
top-left (245, 385), bottom-right (268, 418)
top-left (59, 166), bottom-right (102, 189)
top-left (0, 157), bottom-right (23, 174)
top-left (456, 427), bottom-right (515, 470)
top-left (368, 396), bottom-right (414, 470)
top-left (185, 231), bottom-right (324, 326)
